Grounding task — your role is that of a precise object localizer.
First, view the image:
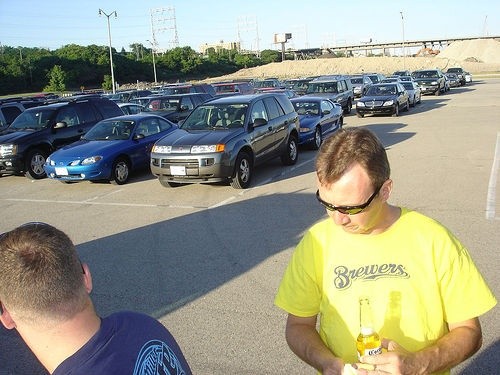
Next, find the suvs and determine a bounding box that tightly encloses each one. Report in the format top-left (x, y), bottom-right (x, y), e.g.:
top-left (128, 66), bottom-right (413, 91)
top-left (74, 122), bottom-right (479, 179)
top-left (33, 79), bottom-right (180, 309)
top-left (0, 95), bottom-right (49, 134)
top-left (150, 91), bottom-right (301, 190)
top-left (446, 67), bottom-right (466, 86)
top-left (0, 95), bottom-right (128, 179)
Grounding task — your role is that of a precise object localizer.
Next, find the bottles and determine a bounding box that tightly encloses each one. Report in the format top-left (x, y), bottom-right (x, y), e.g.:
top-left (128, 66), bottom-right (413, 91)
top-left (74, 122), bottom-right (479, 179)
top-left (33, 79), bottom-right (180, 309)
top-left (357, 322), bottom-right (383, 364)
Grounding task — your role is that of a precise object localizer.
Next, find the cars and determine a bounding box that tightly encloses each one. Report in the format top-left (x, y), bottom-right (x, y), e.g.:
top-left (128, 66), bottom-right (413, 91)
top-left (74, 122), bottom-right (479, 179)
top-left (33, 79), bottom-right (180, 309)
top-left (38, 71), bottom-right (412, 125)
top-left (412, 69), bottom-right (446, 96)
top-left (286, 96), bottom-right (343, 151)
top-left (355, 82), bottom-right (409, 118)
top-left (443, 72), bottom-right (461, 88)
top-left (465, 72), bottom-right (473, 83)
top-left (392, 81), bottom-right (422, 108)
top-left (44, 113), bottom-right (178, 186)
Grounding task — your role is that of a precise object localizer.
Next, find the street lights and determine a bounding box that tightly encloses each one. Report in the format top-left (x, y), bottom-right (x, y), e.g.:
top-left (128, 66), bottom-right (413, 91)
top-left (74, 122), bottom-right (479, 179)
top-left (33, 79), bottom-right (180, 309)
top-left (400, 11), bottom-right (407, 72)
top-left (146, 39), bottom-right (157, 86)
top-left (97, 8), bottom-right (118, 94)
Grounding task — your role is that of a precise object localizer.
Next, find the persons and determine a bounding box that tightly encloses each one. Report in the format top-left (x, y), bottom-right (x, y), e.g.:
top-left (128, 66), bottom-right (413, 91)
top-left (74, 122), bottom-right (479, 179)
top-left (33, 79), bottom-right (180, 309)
top-left (0, 222), bottom-right (193, 375)
top-left (274, 126), bottom-right (499, 375)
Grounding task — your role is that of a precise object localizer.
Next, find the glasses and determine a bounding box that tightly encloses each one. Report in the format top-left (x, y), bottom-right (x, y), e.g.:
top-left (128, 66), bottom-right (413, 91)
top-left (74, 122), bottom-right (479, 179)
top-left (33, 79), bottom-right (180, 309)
top-left (316, 182), bottom-right (384, 216)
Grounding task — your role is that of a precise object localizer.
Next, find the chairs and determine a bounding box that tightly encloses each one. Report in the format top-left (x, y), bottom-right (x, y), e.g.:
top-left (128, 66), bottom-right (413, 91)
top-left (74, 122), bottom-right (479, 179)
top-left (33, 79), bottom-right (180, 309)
top-left (327, 88), bottom-right (335, 91)
top-left (63, 114), bottom-right (74, 126)
top-left (315, 86), bottom-right (322, 92)
top-left (216, 112), bottom-right (230, 126)
top-left (139, 123), bottom-right (150, 135)
